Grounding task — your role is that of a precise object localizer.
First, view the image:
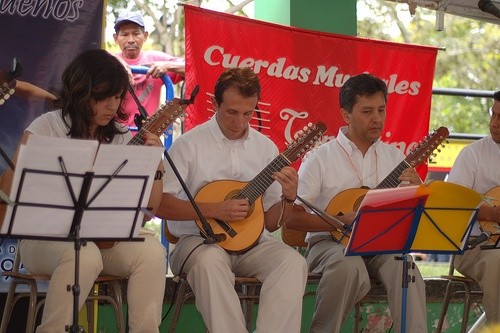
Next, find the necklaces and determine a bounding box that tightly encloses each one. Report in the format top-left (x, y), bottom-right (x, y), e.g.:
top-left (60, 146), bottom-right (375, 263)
top-left (337, 141), bottom-right (379, 188)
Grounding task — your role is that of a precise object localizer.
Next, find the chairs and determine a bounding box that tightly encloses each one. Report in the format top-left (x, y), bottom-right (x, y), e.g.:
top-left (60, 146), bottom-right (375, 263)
top-left (436, 254), bottom-right (484, 333)
top-left (0, 167), bottom-right (125, 333)
top-left (164, 218), bottom-right (261, 333)
top-left (282, 204), bottom-right (388, 333)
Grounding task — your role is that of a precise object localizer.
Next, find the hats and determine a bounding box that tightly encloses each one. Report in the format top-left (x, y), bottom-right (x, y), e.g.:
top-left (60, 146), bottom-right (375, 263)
top-left (114, 11), bottom-right (145, 33)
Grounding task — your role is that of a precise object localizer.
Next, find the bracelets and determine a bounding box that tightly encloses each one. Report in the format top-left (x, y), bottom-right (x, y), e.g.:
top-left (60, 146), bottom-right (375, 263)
top-left (283, 194), bottom-right (297, 203)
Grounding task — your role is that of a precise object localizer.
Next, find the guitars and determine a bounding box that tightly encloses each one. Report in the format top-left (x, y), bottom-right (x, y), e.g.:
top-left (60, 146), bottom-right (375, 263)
top-left (80, 98), bottom-right (187, 249)
top-left (194, 119), bottom-right (330, 255)
top-left (478, 186), bottom-right (500, 244)
top-left (324, 127), bottom-right (451, 243)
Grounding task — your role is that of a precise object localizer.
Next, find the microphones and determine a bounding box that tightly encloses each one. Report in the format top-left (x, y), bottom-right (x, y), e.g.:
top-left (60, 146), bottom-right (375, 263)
top-left (464, 230), bottom-right (491, 252)
top-left (204, 233), bottom-right (226, 244)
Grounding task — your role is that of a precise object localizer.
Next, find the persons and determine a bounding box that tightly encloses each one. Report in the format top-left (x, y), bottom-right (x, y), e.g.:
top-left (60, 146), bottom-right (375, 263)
top-left (446, 91), bottom-right (500, 326)
top-left (284, 74), bottom-right (429, 333)
top-left (109, 12), bottom-right (185, 138)
top-left (0, 49), bottom-right (163, 333)
top-left (154, 64), bottom-right (308, 333)
